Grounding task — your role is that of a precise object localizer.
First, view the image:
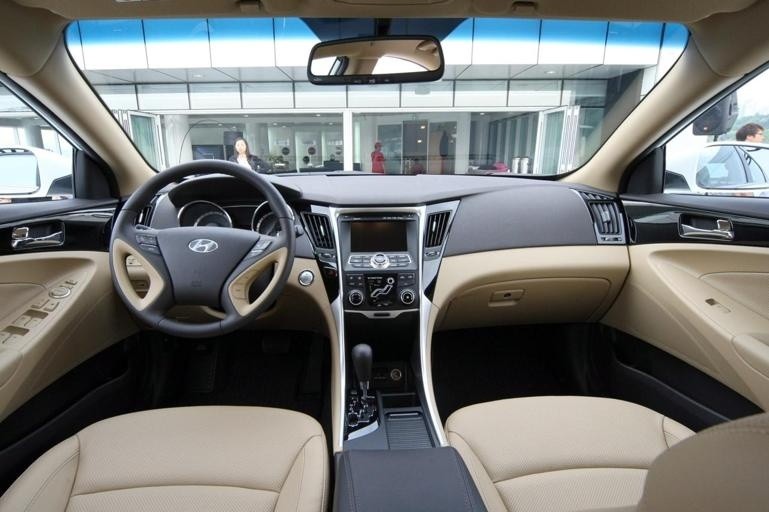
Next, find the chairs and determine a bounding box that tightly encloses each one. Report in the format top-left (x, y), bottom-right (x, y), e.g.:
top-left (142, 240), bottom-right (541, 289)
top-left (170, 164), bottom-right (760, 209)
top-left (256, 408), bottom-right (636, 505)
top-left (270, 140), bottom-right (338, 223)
top-left (444, 396), bottom-right (768, 511)
top-left (0, 406), bottom-right (329, 512)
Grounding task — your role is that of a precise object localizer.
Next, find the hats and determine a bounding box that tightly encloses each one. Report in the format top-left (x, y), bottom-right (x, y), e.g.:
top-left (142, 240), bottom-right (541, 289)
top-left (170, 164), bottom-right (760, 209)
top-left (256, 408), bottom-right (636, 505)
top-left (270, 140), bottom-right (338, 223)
top-left (374, 142), bottom-right (383, 149)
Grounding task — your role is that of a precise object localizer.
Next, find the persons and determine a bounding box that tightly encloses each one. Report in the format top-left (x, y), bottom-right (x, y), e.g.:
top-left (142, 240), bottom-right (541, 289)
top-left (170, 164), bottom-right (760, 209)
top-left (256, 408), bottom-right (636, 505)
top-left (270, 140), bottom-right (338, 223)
top-left (228, 136), bottom-right (270, 174)
top-left (736, 122), bottom-right (765, 144)
top-left (411, 157), bottom-right (424, 175)
top-left (371, 141), bottom-right (385, 174)
